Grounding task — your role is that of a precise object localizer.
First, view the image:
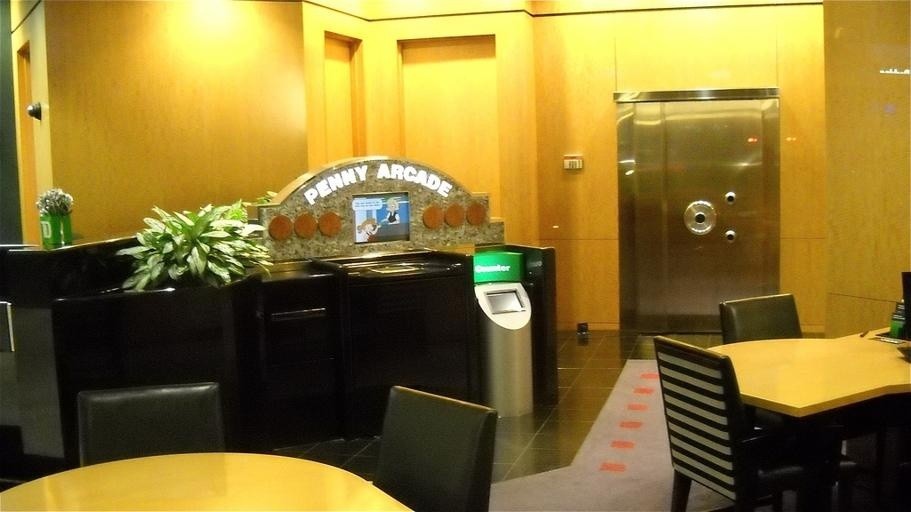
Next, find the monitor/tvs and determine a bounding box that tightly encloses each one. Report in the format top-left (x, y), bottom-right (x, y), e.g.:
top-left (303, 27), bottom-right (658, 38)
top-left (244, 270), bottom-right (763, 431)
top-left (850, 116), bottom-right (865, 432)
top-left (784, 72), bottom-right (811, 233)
top-left (352, 192), bottom-right (409, 244)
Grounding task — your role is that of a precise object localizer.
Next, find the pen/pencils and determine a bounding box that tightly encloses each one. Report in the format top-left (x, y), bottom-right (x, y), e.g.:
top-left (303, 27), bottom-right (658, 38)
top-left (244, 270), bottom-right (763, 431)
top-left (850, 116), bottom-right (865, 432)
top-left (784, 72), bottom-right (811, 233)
top-left (861, 330), bottom-right (869, 337)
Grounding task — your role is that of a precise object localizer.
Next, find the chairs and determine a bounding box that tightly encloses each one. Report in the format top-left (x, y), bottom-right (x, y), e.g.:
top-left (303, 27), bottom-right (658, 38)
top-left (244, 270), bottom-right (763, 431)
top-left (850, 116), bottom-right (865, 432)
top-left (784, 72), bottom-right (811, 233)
top-left (78, 381), bottom-right (225, 465)
top-left (654, 335), bottom-right (824, 509)
top-left (375, 385), bottom-right (497, 512)
top-left (719, 295), bottom-right (813, 343)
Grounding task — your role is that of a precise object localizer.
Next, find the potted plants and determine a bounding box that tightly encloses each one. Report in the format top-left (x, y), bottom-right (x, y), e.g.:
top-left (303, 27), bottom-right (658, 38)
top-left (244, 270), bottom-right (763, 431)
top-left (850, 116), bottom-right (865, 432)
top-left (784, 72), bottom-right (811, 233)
top-left (35, 190), bottom-right (75, 249)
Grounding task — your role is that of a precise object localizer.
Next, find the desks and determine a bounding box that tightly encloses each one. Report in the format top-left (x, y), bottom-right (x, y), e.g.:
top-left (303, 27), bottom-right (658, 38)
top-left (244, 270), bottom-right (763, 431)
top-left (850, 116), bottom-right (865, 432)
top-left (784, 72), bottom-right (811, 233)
top-left (706, 325), bottom-right (911, 509)
top-left (0, 452), bottom-right (412, 511)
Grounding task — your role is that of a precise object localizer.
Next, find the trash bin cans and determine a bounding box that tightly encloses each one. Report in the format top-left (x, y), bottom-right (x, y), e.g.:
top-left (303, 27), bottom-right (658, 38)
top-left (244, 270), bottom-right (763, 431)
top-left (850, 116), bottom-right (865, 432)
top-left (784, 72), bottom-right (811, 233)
top-left (474, 281), bottom-right (533, 418)
